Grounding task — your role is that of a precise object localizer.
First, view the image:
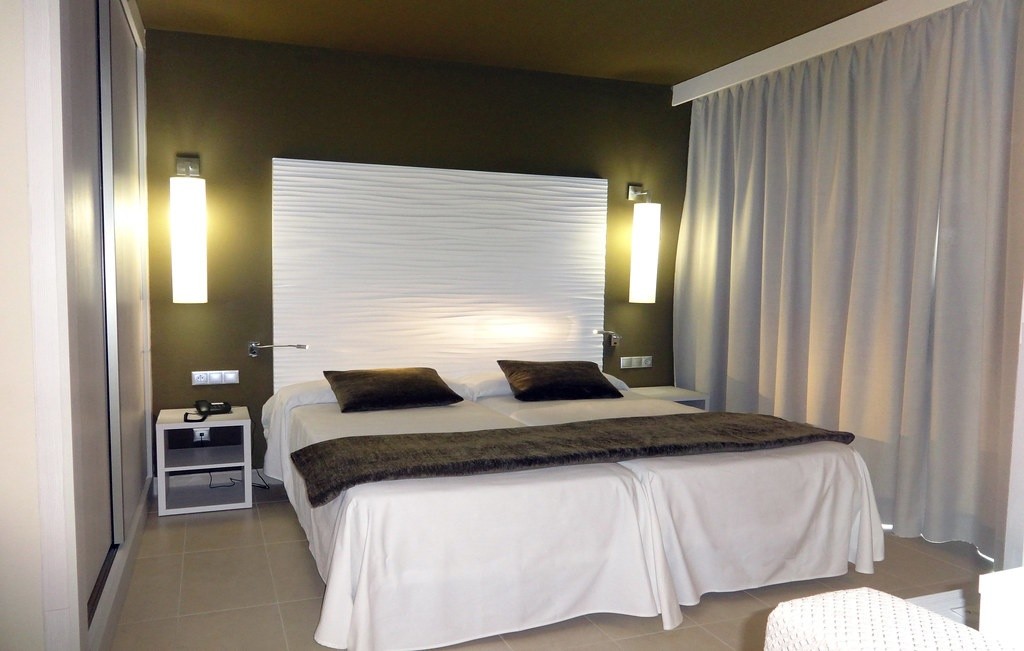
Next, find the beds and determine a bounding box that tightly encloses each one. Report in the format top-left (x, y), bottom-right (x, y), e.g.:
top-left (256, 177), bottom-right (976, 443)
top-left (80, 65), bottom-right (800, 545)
top-left (264, 156), bottom-right (886, 651)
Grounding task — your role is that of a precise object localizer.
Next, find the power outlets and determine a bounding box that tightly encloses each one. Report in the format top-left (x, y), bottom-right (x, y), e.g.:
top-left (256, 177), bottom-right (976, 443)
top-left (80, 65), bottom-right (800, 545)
top-left (191, 370), bottom-right (240, 386)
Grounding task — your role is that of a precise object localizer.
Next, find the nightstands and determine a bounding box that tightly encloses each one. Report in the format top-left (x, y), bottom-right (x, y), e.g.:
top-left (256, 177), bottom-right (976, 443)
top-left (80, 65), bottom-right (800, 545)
top-left (155, 406), bottom-right (253, 518)
top-left (629, 385), bottom-right (710, 412)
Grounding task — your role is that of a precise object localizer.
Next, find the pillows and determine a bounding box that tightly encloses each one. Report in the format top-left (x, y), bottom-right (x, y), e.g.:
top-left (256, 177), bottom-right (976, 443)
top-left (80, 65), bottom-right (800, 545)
top-left (495, 360), bottom-right (625, 402)
top-left (321, 367), bottom-right (465, 414)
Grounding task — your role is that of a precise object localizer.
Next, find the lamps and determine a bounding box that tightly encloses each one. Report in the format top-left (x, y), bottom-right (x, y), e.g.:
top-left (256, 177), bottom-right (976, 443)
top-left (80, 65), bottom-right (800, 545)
top-left (592, 330), bottom-right (620, 347)
top-left (169, 154), bottom-right (210, 304)
top-left (249, 341), bottom-right (309, 358)
top-left (620, 355), bottom-right (653, 369)
top-left (627, 185), bottom-right (661, 304)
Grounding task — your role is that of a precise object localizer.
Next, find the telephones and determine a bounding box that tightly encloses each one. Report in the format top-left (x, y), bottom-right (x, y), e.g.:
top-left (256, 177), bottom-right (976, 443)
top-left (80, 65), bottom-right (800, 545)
top-left (195, 399), bottom-right (231, 415)
